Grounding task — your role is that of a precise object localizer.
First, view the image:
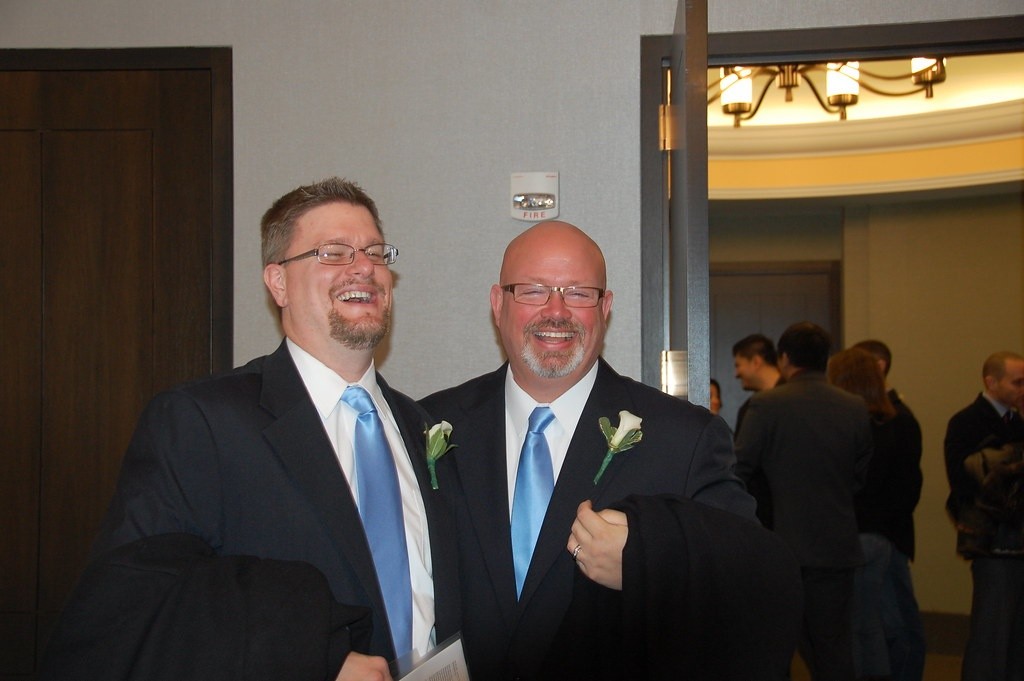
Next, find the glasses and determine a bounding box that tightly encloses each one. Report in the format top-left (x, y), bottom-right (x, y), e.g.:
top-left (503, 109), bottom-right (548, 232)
top-left (277, 243), bottom-right (399, 265)
top-left (500, 283), bottom-right (606, 308)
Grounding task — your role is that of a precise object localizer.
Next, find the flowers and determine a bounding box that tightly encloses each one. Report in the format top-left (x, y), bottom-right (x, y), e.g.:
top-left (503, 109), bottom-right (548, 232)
top-left (424, 421), bottom-right (458, 489)
top-left (593, 411), bottom-right (642, 484)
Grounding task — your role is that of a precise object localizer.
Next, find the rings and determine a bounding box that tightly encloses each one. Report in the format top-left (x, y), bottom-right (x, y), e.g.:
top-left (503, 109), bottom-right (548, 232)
top-left (573, 545), bottom-right (580, 557)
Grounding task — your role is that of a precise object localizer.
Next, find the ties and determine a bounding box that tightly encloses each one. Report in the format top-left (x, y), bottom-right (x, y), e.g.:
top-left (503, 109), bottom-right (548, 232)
top-left (1004, 409), bottom-right (1010, 430)
top-left (510, 406), bottom-right (555, 603)
top-left (339, 386), bottom-right (413, 677)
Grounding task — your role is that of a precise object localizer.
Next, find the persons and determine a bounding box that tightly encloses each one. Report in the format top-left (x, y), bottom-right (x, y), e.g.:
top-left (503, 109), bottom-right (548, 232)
top-left (942, 348), bottom-right (1024, 681)
top-left (709, 378), bottom-right (722, 416)
top-left (44, 181), bottom-right (460, 681)
top-left (419, 219), bottom-right (762, 681)
top-left (734, 322), bottom-right (872, 681)
top-left (828, 340), bottom-right (924, 681)
top-left (732, 333), bottom-right (788, 435)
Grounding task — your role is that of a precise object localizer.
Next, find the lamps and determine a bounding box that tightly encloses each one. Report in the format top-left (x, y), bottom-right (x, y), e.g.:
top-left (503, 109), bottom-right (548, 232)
top-left (706, 57), bottom-right (946, 128)
